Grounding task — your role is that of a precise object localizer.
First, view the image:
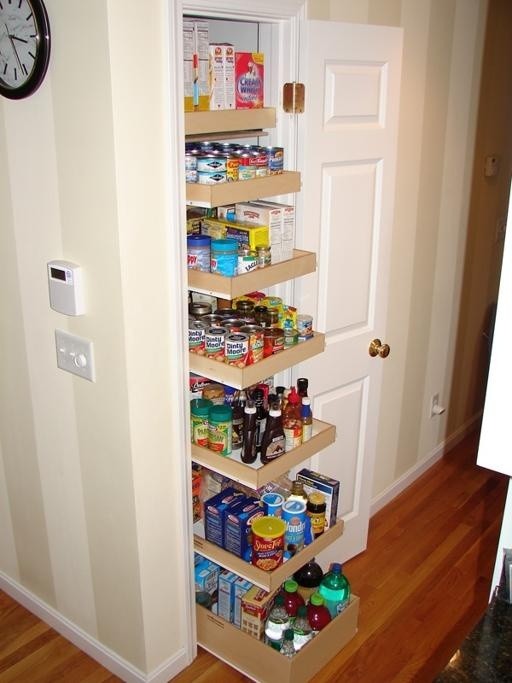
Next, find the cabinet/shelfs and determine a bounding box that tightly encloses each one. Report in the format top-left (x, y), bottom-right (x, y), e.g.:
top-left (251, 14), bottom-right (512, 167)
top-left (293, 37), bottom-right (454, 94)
top-left (177, 2), bottom-right (359, 682)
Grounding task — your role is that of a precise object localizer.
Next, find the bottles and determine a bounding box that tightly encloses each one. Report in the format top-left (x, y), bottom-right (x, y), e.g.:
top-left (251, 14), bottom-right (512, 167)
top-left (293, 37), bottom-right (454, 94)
top-left (265, 557), bottom-right (350, 658)
top-left (240, 378), bottom-right (313, 465)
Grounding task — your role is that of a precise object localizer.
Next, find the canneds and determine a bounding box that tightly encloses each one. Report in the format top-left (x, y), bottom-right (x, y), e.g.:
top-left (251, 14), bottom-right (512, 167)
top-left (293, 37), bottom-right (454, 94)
top-left (256, 244), bottom-right (272, 269)
top-left (185, 142), bottom-right (283, 185)
top-left (188, 292), bottom-right (313, 367)
top-left (306, 492), bottom-right (326, 538)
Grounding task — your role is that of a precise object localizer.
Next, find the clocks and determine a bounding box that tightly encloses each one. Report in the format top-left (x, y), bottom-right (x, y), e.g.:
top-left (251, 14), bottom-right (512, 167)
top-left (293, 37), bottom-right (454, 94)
top-left (0, 0), bottom-right (53, 99)
